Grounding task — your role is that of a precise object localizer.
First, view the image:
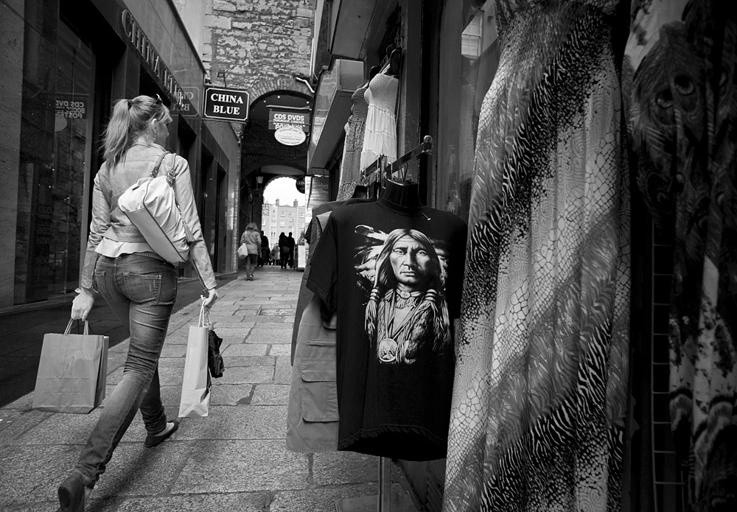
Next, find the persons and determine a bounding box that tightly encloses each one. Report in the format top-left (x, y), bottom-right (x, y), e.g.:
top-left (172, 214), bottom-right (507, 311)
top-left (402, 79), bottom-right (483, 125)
top-left (55, 93), bottom-right (222, 512)
top-left (359, 43), bottom-right (404, 179)
top-left (356, 223), bottom-right (452, 367)
top-left (336, 66), bottom-right (380, 202)
top-left (239, 222), bottom-right (295, 282)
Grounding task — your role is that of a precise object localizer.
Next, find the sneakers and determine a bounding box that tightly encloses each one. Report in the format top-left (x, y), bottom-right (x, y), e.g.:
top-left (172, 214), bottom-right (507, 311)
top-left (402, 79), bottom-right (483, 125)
top-left (56, 474), bottom-right (85, 512)
top-left (144, 420), bottom-right (179, 448)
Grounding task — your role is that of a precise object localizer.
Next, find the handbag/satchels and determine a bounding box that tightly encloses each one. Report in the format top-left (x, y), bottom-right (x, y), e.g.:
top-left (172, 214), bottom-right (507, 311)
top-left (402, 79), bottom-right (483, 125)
top-left (31, 315), bottom-right (110, 415)
top-left (236, 244), bottom-right (248, 260)
top-left (116, 175), bottom-right (192, 266)
top-left (179, 299), bottom-right (212, 417)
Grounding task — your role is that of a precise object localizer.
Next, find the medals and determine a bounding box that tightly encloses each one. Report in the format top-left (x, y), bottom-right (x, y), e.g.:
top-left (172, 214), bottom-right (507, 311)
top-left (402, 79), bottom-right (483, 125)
top-left (377, 336), bottom-right (399, 362)
